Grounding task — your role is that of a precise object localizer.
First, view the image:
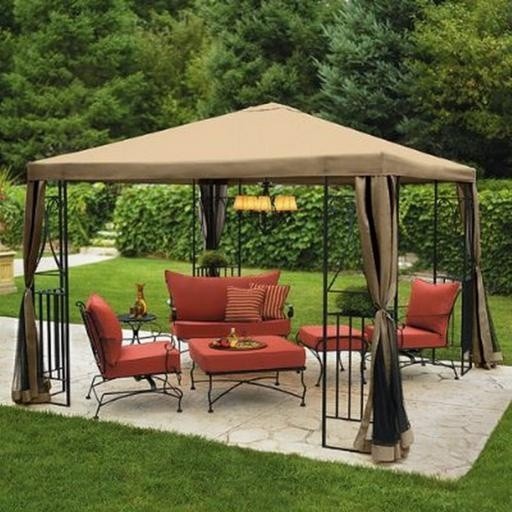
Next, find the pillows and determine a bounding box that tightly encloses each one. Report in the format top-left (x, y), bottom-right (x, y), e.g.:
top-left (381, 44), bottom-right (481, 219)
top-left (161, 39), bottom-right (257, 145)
top-left (249, 282), bottom-right (291, 320)
top-left (406, 279), bottom-right (460, 334)
top-left (86, 293), bottom-right (122, 367)
top-left (224, 286), bottom-right (265, 321)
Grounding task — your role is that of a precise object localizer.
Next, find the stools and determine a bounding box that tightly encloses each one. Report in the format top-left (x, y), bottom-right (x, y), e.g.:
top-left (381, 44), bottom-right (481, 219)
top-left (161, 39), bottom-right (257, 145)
top-left (295, 325), bottom-right (368, 387)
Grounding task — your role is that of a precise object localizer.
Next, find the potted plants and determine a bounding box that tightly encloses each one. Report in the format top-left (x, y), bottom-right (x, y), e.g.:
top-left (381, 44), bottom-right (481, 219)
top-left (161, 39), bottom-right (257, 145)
top-left (196, 250), bottom-right (227, 278)
top-left (0, 164), bottom-right (26, 294)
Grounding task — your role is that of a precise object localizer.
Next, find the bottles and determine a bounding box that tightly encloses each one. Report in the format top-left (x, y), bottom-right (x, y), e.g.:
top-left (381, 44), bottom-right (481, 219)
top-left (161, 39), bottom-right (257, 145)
top-left (128, 280), bottom-right (147, 320)
top-left (227, 328), bottom-right (238, 345)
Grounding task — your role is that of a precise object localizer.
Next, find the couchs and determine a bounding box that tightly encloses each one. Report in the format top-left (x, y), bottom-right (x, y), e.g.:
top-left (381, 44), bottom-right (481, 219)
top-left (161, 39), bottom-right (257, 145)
top-left (164, 269), bottom-right (294, 354)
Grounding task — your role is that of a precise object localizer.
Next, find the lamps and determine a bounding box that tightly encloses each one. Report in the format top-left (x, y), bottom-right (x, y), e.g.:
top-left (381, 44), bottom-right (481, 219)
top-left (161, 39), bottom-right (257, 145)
top-left (232, 177), bottom-right (298, 235)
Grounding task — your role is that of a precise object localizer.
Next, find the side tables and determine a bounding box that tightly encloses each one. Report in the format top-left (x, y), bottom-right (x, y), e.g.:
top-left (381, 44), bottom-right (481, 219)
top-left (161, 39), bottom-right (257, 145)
top-left (118, 314), bottom-right (158, 344)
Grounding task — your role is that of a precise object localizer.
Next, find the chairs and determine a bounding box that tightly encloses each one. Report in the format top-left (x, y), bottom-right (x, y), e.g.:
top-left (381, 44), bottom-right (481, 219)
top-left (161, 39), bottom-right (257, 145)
top-left (75, 293), bottom-right (183, 419)
top-left (366, 278), bottom-right (462, 380)
